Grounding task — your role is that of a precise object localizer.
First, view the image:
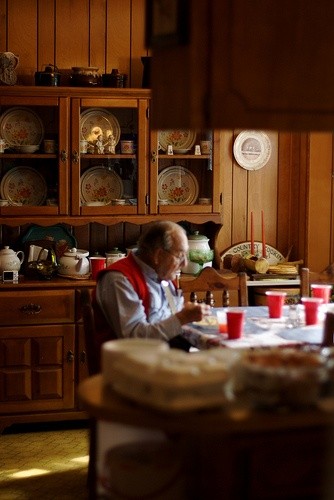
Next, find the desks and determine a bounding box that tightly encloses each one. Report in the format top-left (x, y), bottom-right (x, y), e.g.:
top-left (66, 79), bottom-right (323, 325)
top-left (78, 303), bottom-right (334, 500)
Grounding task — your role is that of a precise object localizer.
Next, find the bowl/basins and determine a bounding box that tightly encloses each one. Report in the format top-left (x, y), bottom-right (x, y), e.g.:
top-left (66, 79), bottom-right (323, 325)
top-left (14, 144), bottom-right (40, 153)
top-left (71, 67), bottom-right (128, 88)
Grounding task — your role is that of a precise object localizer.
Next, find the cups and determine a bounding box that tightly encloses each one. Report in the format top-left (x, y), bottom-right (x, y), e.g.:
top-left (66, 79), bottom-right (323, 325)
top-left (300, 296), bottom-right (323, 325)
top-left (158, 200), bottom-right (168, 206)
top-left (87, 247), bottom-right (138, 280)
top-left (120, 141), bottom-right (134, 154)
top-left (200, 141), bottom-right (211, 154)
top-left (111, 199), bottom-right (125, 206)
top-left (200, 199), bottom-right (211, 205)
top-left (216, 311), bottom-right (227, 334)
top-left (265, 291), bottom-right (288, 318)
top-left (310, 284), bottom-right (332, 305)
top-left (0, 200), bottom-right (10, 205)
top-left (44, 140), bottom-right (55, 154)
top-left (227, 312), bottom-right (244, 338)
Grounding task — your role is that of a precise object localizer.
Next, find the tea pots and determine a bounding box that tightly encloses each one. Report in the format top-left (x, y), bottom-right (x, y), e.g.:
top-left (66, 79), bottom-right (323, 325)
top-left (0, 245), bottom-right (25, 272)
top-left (58, 248), bottom-right (91, 277)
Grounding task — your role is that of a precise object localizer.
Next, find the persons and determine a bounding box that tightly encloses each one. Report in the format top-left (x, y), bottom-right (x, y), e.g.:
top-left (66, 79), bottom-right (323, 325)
top-left (95, 135), bottom-right (104, 155)
top-left (88, 216), bottom-right (212, 375)
top-left (106, 134), bottom-right (116, 154)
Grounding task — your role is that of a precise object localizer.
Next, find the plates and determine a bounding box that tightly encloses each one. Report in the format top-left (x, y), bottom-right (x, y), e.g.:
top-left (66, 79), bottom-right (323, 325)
top-left (232, 130), bottom-right (272, 171)
top-left (231, 338), bottom-right (334, 394)
top-left (0, 105), bottom-right (199, 206)
top-left (221, 241), bottom-right (286, 266)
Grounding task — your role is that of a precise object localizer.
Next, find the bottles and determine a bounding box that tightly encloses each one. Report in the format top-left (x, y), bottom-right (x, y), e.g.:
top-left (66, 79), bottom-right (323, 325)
top-left (181, 230), bottom-right (212, 273)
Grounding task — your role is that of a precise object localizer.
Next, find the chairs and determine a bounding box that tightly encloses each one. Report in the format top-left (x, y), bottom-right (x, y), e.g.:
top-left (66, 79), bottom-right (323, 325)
top-left (71, 289), bottom-right (117, 488)
top-left (173, 267), bottom-right (250, 310)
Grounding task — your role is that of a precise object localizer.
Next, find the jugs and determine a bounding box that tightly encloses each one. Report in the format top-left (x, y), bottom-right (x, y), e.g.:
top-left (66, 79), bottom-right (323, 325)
top-left (35, 63), bottom-right (61, 87)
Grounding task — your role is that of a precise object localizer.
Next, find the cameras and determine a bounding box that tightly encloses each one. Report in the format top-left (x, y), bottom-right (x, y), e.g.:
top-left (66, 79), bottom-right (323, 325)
top-left (3, 270), bottom-right (18, 281)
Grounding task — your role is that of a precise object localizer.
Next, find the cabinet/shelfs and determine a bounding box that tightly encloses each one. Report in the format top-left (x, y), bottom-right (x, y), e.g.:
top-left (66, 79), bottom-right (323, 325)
top-left (0, 85), bottom-right (223, 214)
top-left (0, 278), bottom-right (237, 434)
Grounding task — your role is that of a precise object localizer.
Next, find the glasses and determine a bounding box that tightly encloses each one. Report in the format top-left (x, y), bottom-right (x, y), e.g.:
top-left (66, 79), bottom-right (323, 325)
top-left (163, 250), bottom-right (188, 263)
